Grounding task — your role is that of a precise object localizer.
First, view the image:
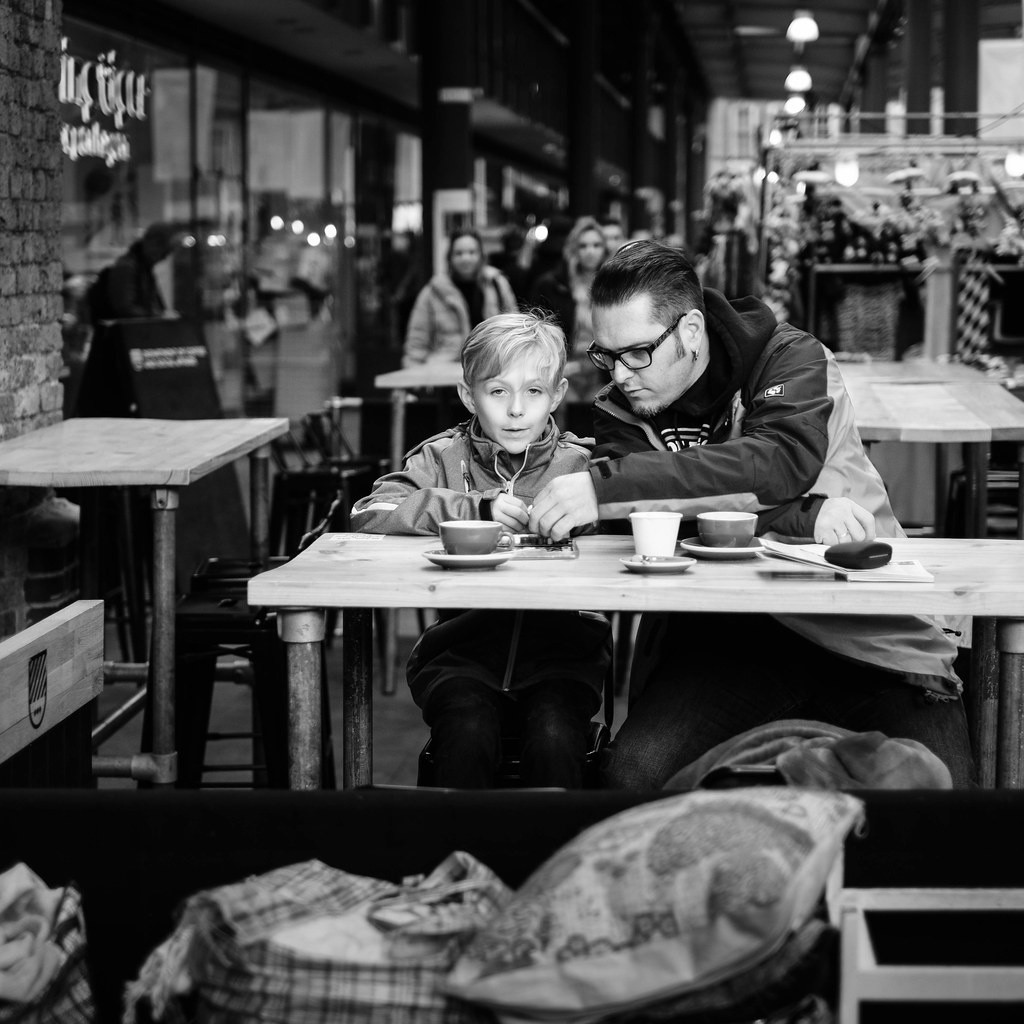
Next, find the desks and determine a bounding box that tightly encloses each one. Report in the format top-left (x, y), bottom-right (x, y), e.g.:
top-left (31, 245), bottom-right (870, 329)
top-left (373, 359), bottom-right (467, 692)
top-left (1, 415), bottom-right (288, 793)
top-left (246, 530), bottom-right (1024, 795)
top-left (839, 361), bottom-right (1023, 537)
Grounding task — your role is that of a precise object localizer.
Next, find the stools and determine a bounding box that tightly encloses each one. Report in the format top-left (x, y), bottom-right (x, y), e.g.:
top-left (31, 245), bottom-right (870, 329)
top-left (162, 389), bottom-right (614, 791)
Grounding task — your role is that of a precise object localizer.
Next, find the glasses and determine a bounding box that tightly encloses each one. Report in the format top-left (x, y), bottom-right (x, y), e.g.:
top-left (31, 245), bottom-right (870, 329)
top-left (586, 311), bottom-right (689, 372)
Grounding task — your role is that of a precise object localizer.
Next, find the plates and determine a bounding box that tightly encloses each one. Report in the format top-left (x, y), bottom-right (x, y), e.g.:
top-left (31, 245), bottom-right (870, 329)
top-left (681, 536), bottom-right (765, 560)
top-left (425, 550), bottom-right (515, 571)
top-left (620, 556), bottom-right (697, 575)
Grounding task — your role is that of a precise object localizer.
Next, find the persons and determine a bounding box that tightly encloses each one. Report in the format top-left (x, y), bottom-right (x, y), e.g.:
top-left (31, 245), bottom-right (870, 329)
top-left (528, 217), bottom-right (610, 439)
top-left (527, 240), bottom-right (974, 790)
top-left (349, 312), bottom-right (603, 790)
top-left (377, 213), bottom-right (711, 372)
top-left (63, 222), bottom-right (175, 416)
top-left (400, 230), bottom-right (523, 440)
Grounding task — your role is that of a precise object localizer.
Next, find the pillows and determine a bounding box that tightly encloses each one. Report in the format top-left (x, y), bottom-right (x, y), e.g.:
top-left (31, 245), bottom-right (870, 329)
top-left (431, 787), bottom-right (862, 1017)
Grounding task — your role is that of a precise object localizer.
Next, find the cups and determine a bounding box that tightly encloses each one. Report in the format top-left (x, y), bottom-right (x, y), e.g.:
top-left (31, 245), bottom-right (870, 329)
top-left (697, 511), bottom-right (758, 547)
top-left (439, 520), bottom-right (514, 554)
top-left (630, 512), bottom-right (683, 556)
